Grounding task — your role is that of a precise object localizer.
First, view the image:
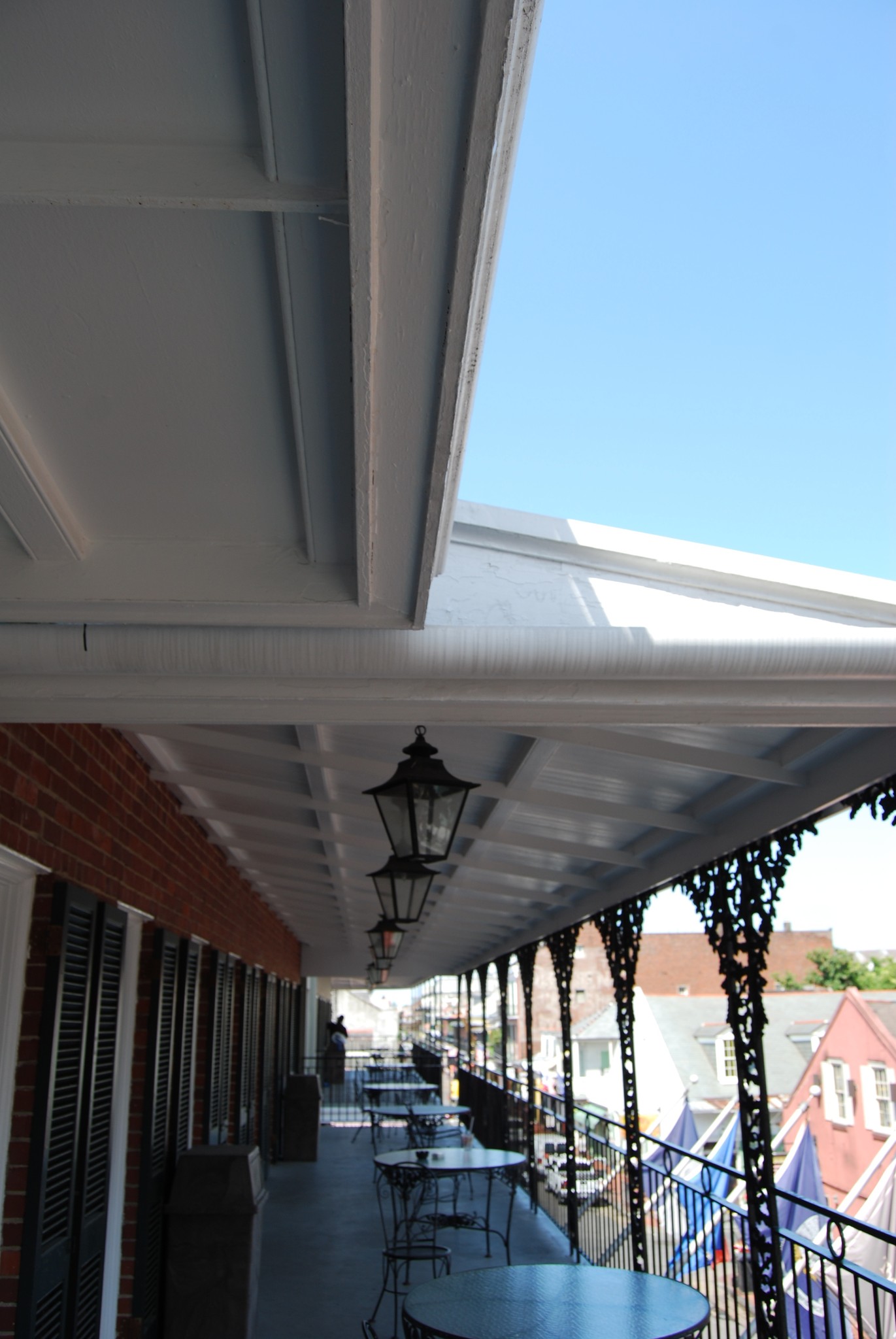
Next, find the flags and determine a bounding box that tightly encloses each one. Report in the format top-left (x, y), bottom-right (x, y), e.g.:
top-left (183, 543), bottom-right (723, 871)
top-left (809, 1154), bottom-right (896, 1339)
top-left (731, 1118), bottom-right (852, 1339)
top-left (651, 1148), bottom-right (706, 1237)
top-left (640, 1099), bottom-right (699, 1222)
top-left (664, 1114), bottom-right (740, 1278)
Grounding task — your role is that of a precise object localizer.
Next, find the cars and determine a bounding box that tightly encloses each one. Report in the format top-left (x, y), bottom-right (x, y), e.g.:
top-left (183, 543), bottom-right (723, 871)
top-left (508, 1117), bottom-right (527, 1142)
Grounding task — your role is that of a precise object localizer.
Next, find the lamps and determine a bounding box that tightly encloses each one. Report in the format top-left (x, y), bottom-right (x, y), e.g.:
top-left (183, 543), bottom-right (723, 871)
top-left (366, 854), bottom-right (441, 922)
top-left (368, 944), bottom-right (394, 971)
top-left (361, 726), bottom-right (481, 860)
top-left (367, 964), bottom-right (389, 984)
top-left (365, 977), bottom-right (378, 991)
top-left (366, 920), bottom-right (408, 959)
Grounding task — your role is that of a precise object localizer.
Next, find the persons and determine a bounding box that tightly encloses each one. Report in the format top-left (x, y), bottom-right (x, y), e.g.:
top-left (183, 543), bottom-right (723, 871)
top-left (320, 1015), bottom-right (348, 1086)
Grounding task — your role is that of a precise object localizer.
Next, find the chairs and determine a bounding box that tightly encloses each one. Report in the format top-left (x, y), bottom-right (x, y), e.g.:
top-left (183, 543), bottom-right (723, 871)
top-left (367, 1160), bottom-right (454, 1339)
top-left (353, 1051), bottom-right (474, 1197)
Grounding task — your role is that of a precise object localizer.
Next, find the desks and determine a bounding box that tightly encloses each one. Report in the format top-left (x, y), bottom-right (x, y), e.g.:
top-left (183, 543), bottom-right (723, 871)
top-left (403, 1263), bottom-right (712, 1339)
top-left (363, 1062), bottom-right (416, 1069)
top-left (371, 1104), bottom-right (470, 1146)
top-left (375, 1148), bottom-right (526, 1265)
top-left (364, 1082), bottom-right (439, 1103)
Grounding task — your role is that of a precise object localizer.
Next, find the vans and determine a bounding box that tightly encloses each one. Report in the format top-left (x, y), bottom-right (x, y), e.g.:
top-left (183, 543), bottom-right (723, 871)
top-left (533, 1133), bottom-right (567, 1177)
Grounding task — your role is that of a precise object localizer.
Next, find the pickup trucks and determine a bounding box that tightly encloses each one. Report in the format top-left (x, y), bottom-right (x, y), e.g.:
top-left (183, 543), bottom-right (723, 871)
top-left (544, 1157), bottom-right (600, 1207)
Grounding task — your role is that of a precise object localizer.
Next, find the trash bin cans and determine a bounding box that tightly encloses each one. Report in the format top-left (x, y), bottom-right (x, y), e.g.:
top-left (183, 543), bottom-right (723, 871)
top-left (164, 1144), bottom-right (269, 1339)
top-left (325, 1043), bottom-right (346, 1084)
top-left (281, 1075), bottom-right (323, 1162)
